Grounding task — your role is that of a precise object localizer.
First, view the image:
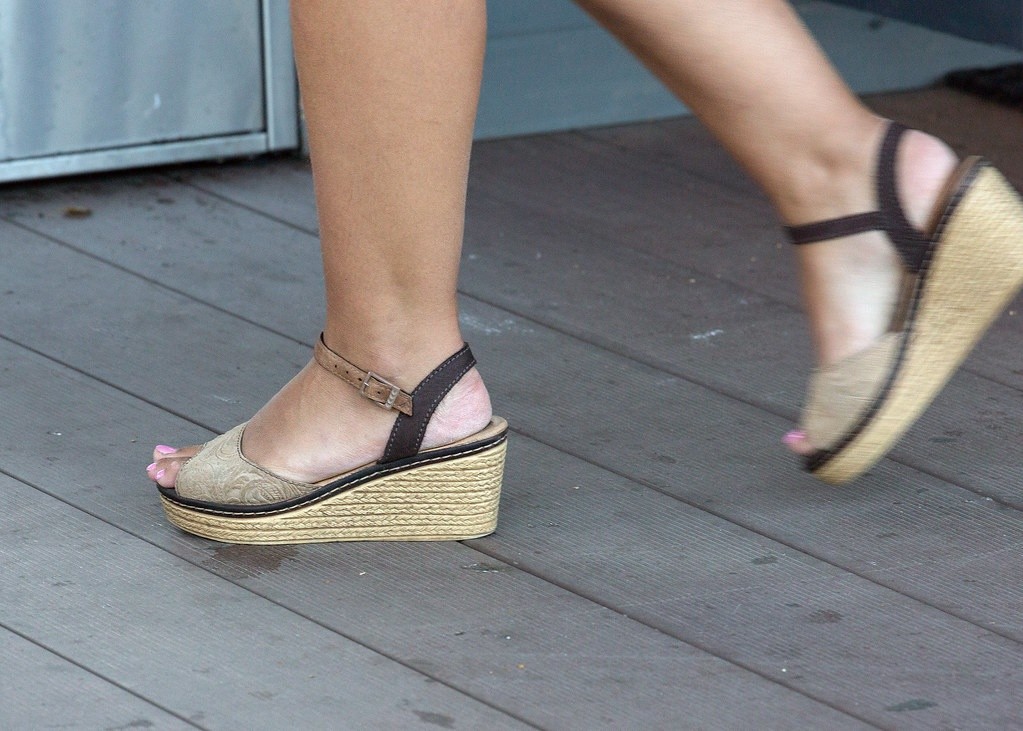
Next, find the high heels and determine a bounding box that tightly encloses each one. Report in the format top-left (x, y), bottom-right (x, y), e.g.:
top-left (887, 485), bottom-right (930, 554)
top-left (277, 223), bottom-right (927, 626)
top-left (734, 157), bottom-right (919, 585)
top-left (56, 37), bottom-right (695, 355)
top-left (150, 332), bottom-right (509, 545)
top-left (782, 121), bottom-right (1023, 486)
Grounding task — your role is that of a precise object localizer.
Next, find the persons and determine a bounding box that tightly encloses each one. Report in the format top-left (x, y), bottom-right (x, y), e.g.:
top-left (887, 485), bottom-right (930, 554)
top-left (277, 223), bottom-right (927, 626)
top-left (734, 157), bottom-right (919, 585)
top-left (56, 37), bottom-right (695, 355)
top-left (143, 0), bottom-right (1022, 545)
